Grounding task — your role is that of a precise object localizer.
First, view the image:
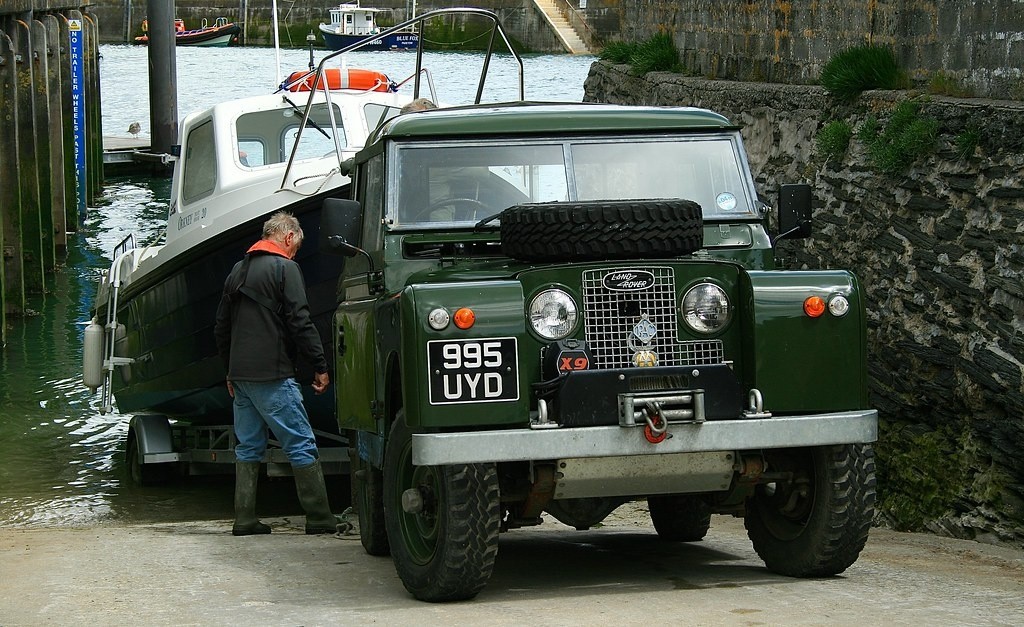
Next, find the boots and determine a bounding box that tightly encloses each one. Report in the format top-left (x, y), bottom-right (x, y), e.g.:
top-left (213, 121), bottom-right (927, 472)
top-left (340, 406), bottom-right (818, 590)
top-left (231, 458), bottom-right (272, 536)
top-left (290, 457), bottom-right (354, 534)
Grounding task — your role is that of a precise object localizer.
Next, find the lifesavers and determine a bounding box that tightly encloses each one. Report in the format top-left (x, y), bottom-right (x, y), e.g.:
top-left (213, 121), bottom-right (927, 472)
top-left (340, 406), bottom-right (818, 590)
top-left (289, 68), bottom-right (389, 92)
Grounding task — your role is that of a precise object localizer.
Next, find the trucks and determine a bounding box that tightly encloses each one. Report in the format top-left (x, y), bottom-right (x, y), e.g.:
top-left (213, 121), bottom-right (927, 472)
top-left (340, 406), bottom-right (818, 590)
top-left (127, 6), bottom-right (880, 603)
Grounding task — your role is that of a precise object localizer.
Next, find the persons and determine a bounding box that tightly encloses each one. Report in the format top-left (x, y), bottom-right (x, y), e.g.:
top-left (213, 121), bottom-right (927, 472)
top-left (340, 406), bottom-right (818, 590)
top-left (219, 212), bottom-right (352, 535)
top-left (399, 98), bottom-right (488, 221)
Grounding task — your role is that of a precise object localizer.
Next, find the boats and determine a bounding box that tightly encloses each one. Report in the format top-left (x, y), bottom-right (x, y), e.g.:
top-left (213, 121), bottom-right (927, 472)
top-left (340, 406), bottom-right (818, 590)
top-left (134, 16), bottom-right (241, 47)
top-left (80, 70), bottom-right (539, 422)
top-left (319, 4), bottom-right (419, 51)
